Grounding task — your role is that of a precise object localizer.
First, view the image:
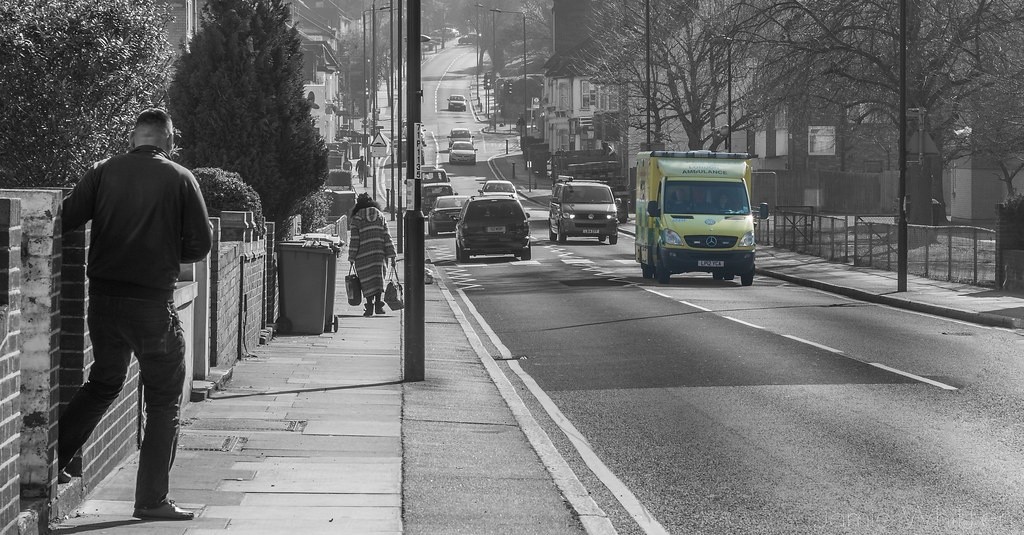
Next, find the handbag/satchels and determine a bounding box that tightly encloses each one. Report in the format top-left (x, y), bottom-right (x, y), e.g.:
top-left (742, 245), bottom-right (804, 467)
top-left (345, 265), bottom-right (362, 306)
top-left (385, 266), bottom-right (404, 310)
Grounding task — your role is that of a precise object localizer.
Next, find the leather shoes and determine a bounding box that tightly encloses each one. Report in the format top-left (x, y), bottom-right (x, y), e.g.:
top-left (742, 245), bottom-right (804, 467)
top-left (133, 498), bottom-right (194, 521)
top-left (59, 470), bottom-right (72, 484)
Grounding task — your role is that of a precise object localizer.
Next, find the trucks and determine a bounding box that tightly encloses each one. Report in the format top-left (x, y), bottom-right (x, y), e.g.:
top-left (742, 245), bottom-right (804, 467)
top-left (550, 149), bottom-right (629, 224)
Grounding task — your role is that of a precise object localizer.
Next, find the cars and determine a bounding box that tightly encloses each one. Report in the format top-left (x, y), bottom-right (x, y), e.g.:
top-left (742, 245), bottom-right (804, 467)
top-left (478, 179), bottom-right (518, 198)
top-left (394, 140), bottom-right (426, 165)
top-left (447, 128), bottom-right (475, 147)
top-left (421, 165), bottom-right (451, 183)
top-left (457, 33), bottom-right (481, 44)
top-left (421, 183), bottom-right (458, 216)
top-left (447, 95), bottom-right (467, 111)
top-left (427, 195), bottom-right (471, 236)
top-left (447, 140), bottom-right (478, 165)
top-left (421, 27), bottom-right (459, 53)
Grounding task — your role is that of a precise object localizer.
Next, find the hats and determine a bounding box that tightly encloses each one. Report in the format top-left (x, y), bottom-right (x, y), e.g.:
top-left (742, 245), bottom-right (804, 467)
top-left (357, 192), bottom-right (372, 203)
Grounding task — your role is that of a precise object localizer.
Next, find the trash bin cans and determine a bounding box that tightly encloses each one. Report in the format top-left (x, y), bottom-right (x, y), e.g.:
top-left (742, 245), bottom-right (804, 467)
top-left (351, 142), bottom-right (361, 159)
top-left (275, 235), bottom-right (346, 335)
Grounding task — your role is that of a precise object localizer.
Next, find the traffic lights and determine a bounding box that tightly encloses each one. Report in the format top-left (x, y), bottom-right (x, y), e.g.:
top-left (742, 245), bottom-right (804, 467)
top-left (504, 80), bottom-right (513, 96)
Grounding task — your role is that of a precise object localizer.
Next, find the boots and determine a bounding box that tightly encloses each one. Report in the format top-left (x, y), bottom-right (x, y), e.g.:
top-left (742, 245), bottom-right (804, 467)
top-left (364, 304), bottom-right (374, 317)
top-left (374, 302), bottom-right (386, 314)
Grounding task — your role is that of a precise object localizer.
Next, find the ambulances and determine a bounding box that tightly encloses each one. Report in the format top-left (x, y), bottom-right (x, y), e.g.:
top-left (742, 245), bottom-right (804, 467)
top-left (634, 149), bottom-right (770, 287)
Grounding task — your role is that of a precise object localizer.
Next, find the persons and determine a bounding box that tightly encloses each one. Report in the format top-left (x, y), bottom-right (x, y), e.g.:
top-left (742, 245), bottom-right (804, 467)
top-left (343, 158), bottom-right (352, 171)
top-left (710, 192), bottom-right (735, 212)
top-left (355, 156), bottom-right (366, 184)
top-left (348, 194), bottom-right (397, 317)
top-left (58, 108), bottom-right (213, 522)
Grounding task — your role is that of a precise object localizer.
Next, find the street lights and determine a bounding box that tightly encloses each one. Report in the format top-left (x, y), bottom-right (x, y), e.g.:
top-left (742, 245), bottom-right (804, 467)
top-left (708, 34), bottom-right (733, 153)
top-left (362, 6), bottom-right (390, 201)
top-left (475, 4), bottom-right (484, 101)
top-left (489, 7), bottom-right (527, 135)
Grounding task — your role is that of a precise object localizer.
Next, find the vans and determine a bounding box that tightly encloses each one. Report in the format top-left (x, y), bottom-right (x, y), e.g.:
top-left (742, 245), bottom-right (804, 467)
top-left (548, 181), bottom-right (621, 246)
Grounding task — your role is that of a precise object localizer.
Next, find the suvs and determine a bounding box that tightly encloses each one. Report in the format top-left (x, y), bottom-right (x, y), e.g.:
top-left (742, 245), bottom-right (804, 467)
top-left (450, 195), bottom-right (531, 262)
